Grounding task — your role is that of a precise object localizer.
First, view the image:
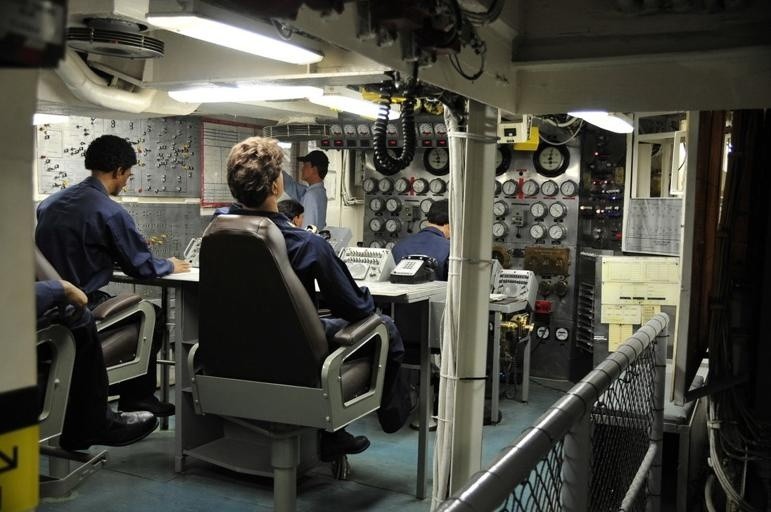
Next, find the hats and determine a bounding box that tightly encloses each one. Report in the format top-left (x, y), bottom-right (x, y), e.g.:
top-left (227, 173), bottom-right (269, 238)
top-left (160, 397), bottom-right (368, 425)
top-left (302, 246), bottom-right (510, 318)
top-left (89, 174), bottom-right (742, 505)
top-left (296, 150), bottom-right (328, 166)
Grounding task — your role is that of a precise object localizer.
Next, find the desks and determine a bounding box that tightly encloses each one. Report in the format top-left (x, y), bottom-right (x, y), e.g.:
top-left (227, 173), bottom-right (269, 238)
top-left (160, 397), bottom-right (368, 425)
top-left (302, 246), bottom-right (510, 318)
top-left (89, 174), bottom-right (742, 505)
top-left (483, 298), bottom-right (533, 427)
top-left (102, 257), bottom-right (450, 506)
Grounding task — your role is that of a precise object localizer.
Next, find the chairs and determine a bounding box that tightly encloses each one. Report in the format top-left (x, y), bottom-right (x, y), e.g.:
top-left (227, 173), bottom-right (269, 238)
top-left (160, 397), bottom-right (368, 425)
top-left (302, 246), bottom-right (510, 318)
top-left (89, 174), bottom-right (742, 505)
top-left (35, 246), bottom-right (160, 478)
top-left (36, 289), bottom-right (111, 502)
top-left (181, 209), bottom-right (394, 512)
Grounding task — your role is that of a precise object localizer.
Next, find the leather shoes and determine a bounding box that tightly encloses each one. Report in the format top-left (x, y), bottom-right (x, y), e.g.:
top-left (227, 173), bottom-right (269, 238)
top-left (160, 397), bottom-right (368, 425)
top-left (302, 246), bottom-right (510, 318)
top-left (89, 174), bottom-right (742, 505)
top-left (117, 397), bottom-right (176, 419)
top-left (316, 429), bottom-right (371, 463)
top-left (377, 386), bottom-right (416, 434)
top-left (58, 407), bottom-right (159, 452)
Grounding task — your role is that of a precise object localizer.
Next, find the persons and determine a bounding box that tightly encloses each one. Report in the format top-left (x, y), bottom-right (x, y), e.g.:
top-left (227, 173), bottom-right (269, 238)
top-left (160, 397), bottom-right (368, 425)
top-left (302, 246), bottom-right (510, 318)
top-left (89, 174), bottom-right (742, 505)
top-left (391, 199), bottom-right (451, 282)
top-left (35, 134), bottom-right (190, 416)
top-left (207, 134), bottom-right (419, 462)
top-left (35, 280), bottom-right (159, 453)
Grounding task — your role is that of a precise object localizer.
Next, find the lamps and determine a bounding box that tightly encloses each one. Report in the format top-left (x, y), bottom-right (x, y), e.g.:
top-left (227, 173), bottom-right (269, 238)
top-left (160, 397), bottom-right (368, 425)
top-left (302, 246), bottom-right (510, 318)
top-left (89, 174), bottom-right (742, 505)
top-left (567, 109), bottom-right (636, 138)
top-left (145, 0), bottom-right (327, 70)
top-left (308, 84), bottom-right (403, 125)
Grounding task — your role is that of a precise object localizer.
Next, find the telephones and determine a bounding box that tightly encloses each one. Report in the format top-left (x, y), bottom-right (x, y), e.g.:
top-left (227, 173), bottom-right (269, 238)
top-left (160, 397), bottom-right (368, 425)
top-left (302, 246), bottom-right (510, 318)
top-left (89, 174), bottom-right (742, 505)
top-left (390, 255), bottom-right (438, 284)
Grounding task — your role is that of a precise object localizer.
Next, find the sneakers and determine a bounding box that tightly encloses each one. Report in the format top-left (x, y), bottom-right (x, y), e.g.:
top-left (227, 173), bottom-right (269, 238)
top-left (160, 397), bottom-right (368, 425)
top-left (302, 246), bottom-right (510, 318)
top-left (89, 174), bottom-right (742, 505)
top-left (484, 407), bottom-right (502, 427)
